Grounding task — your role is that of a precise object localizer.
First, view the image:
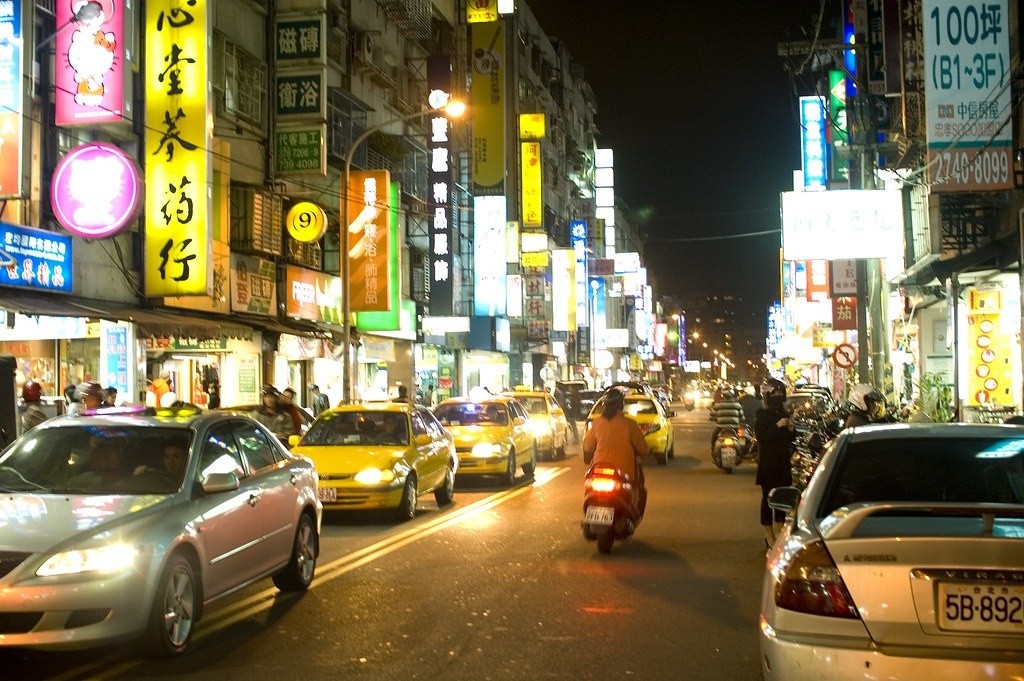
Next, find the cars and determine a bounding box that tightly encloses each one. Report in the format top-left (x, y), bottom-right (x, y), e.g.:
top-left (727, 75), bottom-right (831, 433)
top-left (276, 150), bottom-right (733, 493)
top-left (584, 395), bottom-right (678, 466)
top-left (759, 423), bottom-right (1024, 681)
top-left (431, 395), bottom-right (539, 485)
top-left (494, 391), bottom-right (567, 463)
top-left (286, 402), bottom-right (459, 519)
top-left (0, 412), bottom-right (323, 657)
top-left (554, 377), bottom-right (672, 421)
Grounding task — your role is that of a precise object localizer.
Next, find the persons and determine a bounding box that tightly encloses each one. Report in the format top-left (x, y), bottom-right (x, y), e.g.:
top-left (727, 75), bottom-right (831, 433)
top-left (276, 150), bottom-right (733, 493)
top-left (312, 385), bottom-right (330, 417)
top-left (581, 389), bottom-right (652, 483)
top-left (393, 385), bottom-right (412, 403)
top-left (64, 382), bottom-right (117, 414)
top-left (160, 391), bottom-right (199, 409)
top-left (680, 382), bottom-right (717, 401)
top-left (18, 381), bottom-right (48, 433)
top-left (755, 380), bottom-right (796, 550)
top-left (251, 385), bottom-right (301, 450)
top-left (846, 384), bottom-right (887, 428)
top-left (904, 381), bottom-right (956, 423)
top-left (205, 383), bottom-right (221, 410)
top-left (707, 383), bottom-right (765, 465)
top-left (545, 386), bottom-right (579, 444)
top-left (148, 373), bottom-right (173, 407)
top-left (416, 383), bottom-right (433, 405)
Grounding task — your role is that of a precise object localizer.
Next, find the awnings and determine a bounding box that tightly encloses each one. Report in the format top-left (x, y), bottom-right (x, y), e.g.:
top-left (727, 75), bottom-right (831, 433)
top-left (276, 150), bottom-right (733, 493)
top-left (0, 294), bottom-right (255, 342)
top-left (234, 312), bottom-right (362, 362)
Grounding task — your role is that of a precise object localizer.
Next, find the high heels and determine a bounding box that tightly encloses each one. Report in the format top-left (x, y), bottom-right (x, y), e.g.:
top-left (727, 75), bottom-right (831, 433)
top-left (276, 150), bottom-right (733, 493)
top-left (764, 537), bottom-right (772, 550)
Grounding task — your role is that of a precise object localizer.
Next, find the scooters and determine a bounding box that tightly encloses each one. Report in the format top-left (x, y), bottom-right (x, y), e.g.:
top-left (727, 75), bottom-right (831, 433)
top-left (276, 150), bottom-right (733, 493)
top-left (784, 383), bottom-right (843, 491)
top-left (713, 424), bottom-right (746, 473)
top-left (578, 423), bottom-right (661, 554)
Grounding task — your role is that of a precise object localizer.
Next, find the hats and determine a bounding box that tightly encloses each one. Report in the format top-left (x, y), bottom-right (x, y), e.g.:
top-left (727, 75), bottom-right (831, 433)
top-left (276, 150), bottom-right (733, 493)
top-left (161, 371), bottom-right (170, 379)
top-left (308, 384), bottom-right (318, 391)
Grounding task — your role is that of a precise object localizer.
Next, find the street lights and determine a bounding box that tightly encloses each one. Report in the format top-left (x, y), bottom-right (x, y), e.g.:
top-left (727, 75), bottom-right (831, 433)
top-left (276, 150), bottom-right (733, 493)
top-left (341, 101), bottom-right (468, 403)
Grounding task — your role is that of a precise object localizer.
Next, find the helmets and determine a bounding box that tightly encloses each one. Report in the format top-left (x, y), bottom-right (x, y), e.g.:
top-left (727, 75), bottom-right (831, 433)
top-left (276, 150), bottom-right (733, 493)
top-left (848, 384), bottom-right (884, 412)
top-left (603, 388), bottom-right (624, 406)
top-left (78, 381), bottom-right (103, 402)
top-left (722, 388), bottom-right (735, 398)
top-left (261, 387), bottom-right (279, 404)
top-left (23, 381), bottom-right (42, 401)
